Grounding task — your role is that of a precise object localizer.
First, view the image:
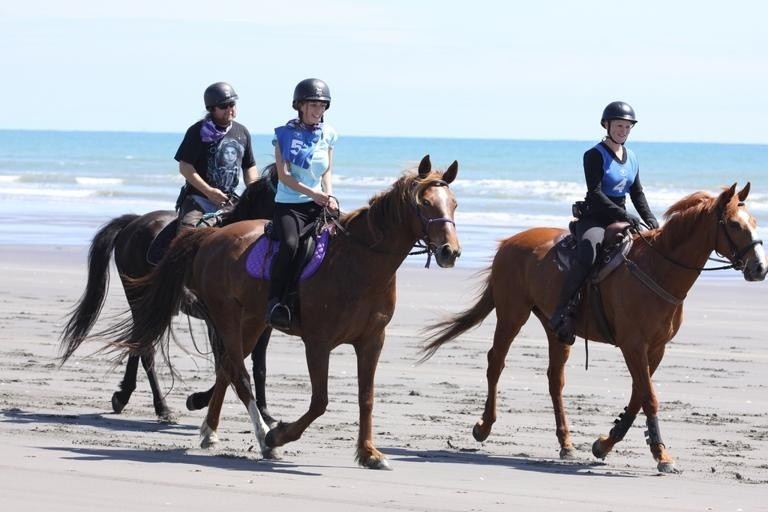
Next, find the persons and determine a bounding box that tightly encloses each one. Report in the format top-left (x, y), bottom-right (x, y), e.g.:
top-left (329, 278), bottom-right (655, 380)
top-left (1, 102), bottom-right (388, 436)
top-left (555, 102), bottom-right (659, 346)
top-left (175, 81), bottom-right (259, 304)
top-left (214, 137), bottom-right (242, 185)
top-left (269, 77), bottom-right (340, 332)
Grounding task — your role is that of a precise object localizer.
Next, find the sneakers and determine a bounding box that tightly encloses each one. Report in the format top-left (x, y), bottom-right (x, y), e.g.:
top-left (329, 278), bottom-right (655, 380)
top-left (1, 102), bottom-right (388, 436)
top-left (265, 296), bottom-right (291, 331)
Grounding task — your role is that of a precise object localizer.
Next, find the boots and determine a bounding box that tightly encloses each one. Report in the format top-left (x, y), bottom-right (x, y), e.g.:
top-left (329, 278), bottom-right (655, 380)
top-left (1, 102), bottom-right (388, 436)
top-left (547, 258), bottom-right (591, 346)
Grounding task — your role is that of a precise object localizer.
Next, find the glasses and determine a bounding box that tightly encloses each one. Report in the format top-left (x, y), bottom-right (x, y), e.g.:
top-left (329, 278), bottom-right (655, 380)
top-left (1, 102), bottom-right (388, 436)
top-left (211, 101), bottom-right (235, 110)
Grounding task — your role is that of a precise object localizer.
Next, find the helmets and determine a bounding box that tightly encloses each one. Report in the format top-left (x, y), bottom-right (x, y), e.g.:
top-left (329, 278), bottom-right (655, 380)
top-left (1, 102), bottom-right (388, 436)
top-left (203, 82), bottom-right (239, 112)
top-left (600, 101), bottom-right (638, 130)
top-left (292, 78), bottom-right (331, 111)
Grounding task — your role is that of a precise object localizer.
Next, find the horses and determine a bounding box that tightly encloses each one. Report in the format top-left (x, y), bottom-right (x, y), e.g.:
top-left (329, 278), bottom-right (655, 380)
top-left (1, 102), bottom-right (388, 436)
top-left (413, 181), bottom-right (768, 474)
top-left (79, 154), bottom-right (462, 473)
top-left (56, 163), bottom-right (278, 430)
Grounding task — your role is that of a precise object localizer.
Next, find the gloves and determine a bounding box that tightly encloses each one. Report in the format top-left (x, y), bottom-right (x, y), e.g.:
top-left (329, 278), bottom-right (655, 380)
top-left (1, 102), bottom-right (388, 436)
top-left (626, 213), bottom-right (640, 228)
top-left (645, 218), bottom-right (659, 230)
